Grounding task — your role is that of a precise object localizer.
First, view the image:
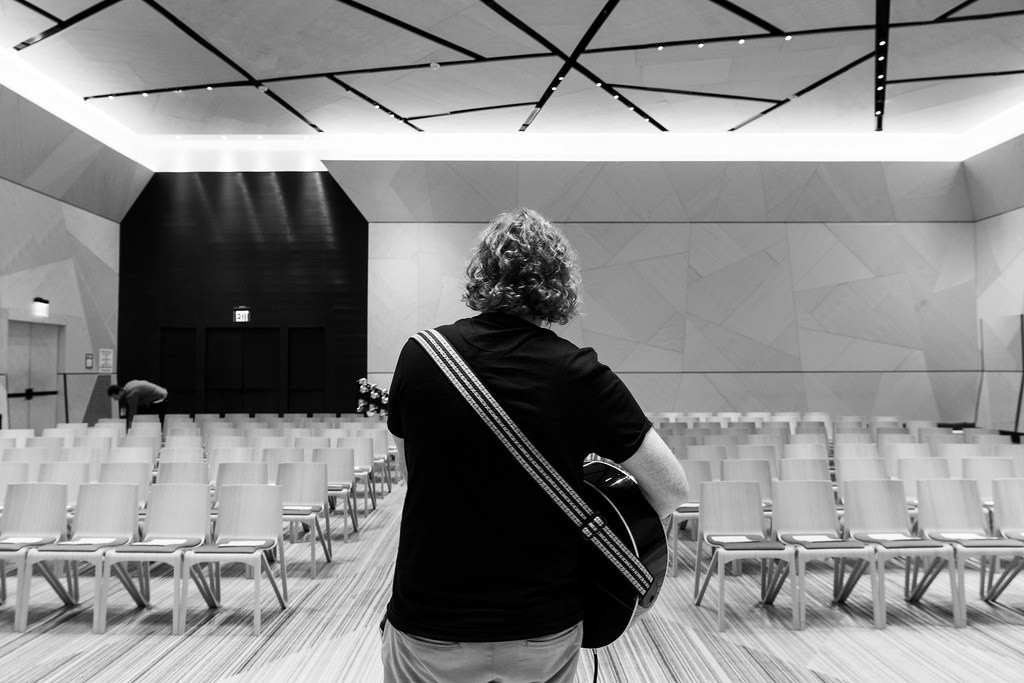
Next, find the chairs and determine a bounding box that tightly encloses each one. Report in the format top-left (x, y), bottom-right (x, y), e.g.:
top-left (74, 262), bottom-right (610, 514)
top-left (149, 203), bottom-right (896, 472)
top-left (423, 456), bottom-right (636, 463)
top-left (643, 410), bottom-right (1024, 633)
top-left (1, 411), bottom-right (400, 633)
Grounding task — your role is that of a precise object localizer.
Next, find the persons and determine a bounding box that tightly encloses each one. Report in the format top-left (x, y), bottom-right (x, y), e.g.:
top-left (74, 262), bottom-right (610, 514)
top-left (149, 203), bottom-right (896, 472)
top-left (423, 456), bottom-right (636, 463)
top-left (107, 378), bottom-right (171, 437)
top-left (372, 208), bottom-right (693, 683)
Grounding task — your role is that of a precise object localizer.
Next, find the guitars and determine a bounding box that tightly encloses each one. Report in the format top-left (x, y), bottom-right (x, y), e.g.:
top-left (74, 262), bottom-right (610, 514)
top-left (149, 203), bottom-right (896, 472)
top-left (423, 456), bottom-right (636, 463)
top-left (354, 376), bottom-right (669, 649)
